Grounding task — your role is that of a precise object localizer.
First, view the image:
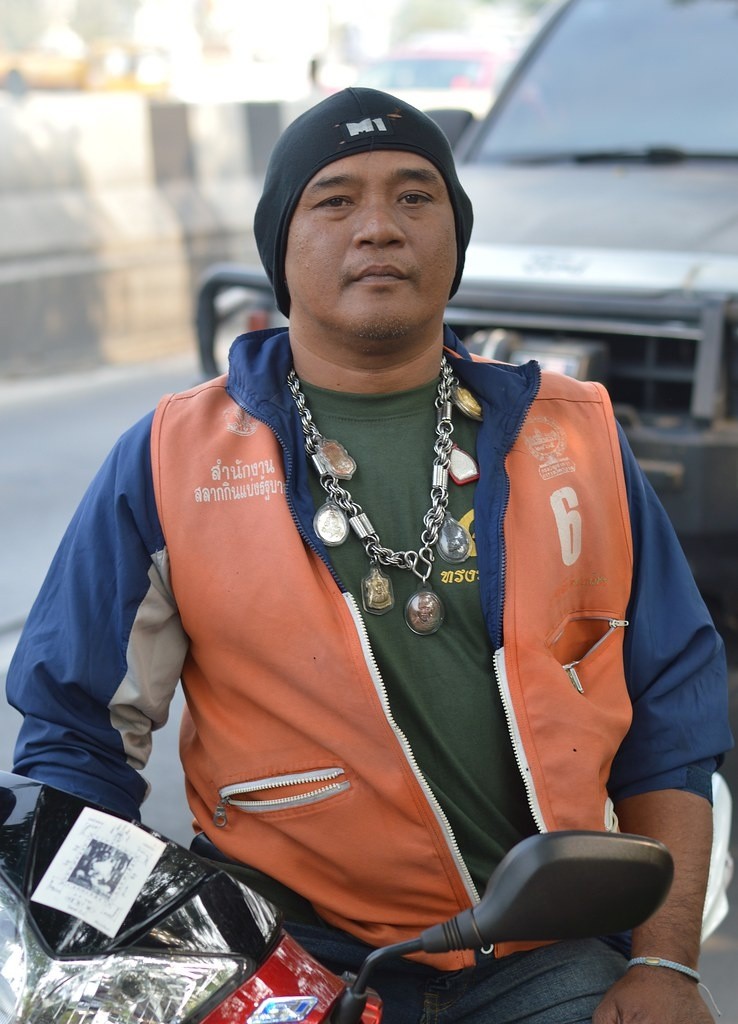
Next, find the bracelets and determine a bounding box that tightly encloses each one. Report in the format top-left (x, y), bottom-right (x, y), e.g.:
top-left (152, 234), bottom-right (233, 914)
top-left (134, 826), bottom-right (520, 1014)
top-left (624, 956), bottom-right (702, 987)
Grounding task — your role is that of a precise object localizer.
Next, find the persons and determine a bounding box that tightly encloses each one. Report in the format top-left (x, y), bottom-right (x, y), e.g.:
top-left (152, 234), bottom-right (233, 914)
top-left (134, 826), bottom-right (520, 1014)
top-left (7, 87), bottom-right (738, 1024)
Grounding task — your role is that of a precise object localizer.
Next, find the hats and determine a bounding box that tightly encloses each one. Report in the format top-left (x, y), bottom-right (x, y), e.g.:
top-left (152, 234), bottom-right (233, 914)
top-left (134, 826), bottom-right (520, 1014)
top-left (253, 86), bottom-right (473, 320)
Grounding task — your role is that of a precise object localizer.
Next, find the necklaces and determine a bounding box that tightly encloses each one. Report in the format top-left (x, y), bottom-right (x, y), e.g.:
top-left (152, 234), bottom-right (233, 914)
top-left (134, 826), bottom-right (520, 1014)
top-left (287, 353), bottom-right (486, 637)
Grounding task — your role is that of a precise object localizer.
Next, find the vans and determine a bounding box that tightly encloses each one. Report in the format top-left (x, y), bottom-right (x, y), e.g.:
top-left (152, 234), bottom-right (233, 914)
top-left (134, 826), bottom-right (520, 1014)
top-left (193, 0), bottom-right (738, 680)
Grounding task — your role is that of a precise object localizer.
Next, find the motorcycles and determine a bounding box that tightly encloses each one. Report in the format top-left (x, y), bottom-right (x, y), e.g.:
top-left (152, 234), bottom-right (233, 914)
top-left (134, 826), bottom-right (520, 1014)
top-left (0, 754), bottom-right (675, 1024)
